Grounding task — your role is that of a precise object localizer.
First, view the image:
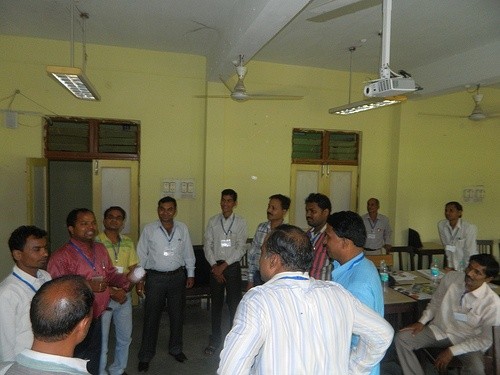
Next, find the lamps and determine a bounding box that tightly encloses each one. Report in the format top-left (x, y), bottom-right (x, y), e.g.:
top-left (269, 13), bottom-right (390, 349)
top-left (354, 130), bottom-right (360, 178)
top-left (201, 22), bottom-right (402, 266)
top-left (46, 2), bottom-right (102, 101)
top-left (327, 30), bottom-right (405, 115)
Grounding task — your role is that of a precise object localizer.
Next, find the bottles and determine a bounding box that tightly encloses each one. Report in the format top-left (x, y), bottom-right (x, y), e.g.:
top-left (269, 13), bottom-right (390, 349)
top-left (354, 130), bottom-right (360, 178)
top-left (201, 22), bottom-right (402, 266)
top-left (379, 263), bottom-right (388, 292)
top-left (430, 264), bottom-right (440, 287)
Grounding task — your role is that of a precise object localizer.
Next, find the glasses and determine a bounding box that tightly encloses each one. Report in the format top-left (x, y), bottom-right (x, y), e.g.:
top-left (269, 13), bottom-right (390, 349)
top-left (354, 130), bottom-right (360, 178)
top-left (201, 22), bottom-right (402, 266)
top-left (223, 199), bottom-right (232, 202)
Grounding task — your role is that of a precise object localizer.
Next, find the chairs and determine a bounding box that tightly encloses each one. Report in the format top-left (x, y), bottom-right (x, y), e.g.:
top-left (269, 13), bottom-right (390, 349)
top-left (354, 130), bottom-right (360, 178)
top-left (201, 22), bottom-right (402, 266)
top-left (183, 246), bottom-right (212, 312)
top-left (477, 241), bottom-right (492, 257)
top-left (387, 246), bottom-right (449, 271)
top-left (239, 239), bottom-right (254, 290)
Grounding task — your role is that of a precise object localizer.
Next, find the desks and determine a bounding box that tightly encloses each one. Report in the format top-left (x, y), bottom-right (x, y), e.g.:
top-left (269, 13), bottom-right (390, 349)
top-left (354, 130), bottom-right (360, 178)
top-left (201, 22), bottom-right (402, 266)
top-left (370, 268), bottom-right (500, 329)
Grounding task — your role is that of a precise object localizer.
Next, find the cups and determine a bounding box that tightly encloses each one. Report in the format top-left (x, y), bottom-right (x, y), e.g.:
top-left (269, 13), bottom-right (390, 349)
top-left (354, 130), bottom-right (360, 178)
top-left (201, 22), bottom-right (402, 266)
top-left (92, 276), bottom-right (106, 292)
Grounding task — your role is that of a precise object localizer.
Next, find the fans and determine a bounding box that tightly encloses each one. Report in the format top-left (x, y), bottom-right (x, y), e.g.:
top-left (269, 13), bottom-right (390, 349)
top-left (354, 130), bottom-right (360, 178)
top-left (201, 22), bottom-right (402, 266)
top-left (193, 53), bottom-right (304, 105)
top-left (417, 83), bottom-right (499, 122)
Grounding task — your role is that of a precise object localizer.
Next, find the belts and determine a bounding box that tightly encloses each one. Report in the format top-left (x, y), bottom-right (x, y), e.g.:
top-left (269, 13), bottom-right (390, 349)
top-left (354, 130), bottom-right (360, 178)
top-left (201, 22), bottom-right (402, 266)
top-left (219, 260), bottom-right (225, 264)
top-left (153, 268), bottom-right (182, 275)
top-left (364, 248), bottom-right (376, 251)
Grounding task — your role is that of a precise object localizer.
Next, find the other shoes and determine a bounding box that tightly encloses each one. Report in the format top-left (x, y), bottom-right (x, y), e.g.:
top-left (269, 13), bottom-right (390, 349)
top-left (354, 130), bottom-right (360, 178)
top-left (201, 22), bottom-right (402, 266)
top-left (204, 343), bottom-right (219, 355)
top-left (169, 352), bottom-right (188, 363)
top-left (122, 371), bottom-right (128, 375)
top-left (138, 362), bottom-right (149, 374)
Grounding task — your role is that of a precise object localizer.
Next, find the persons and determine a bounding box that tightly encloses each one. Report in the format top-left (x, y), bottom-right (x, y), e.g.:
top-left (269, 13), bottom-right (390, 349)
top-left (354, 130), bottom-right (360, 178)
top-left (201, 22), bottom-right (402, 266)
top-left (217, 223), bottom-right (395, 375)
top-left (204, 189), bottom-right (247, 353)
top-left (100, 206), bottom-right (141, 375)
top-left (322, 211), bottom-right (384, 375)
top-left (438, 201), bottom-right (477, 271)
top-left (305, 193), bottom-right (332, 281)
top-left (47, 208), bottom-right (133, 375)
top-left (135, 196), bottom-right (196, 374)
top-left (361, 198), bottom-right (391, 256)
top-left (394, 254), bottom-right (500, 375)
top-left (248, 194), bottom-right (292, 289)
top-left (0, 274), bottom-right (94, 375)
top-left (0, 225), bottom-right (52, 362)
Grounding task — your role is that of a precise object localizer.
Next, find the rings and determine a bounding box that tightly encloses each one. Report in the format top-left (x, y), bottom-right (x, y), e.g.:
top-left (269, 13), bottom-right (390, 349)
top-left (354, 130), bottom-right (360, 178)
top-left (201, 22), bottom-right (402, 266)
top-left (138, 290), bottom-right (141, 292)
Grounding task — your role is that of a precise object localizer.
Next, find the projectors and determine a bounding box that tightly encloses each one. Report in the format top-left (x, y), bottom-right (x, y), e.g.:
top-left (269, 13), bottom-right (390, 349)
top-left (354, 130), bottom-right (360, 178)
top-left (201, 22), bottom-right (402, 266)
top-left (363, 76), bottom-right (416, 98)
